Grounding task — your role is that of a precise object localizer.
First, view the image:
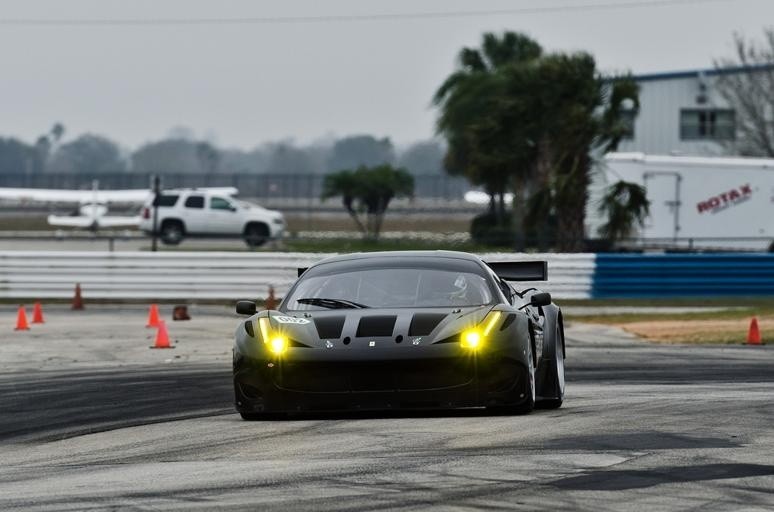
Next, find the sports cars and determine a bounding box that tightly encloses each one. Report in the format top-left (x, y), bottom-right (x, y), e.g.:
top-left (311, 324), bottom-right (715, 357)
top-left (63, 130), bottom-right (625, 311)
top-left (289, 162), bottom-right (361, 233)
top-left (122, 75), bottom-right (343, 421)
top-left (232, 249), bottom-right (566, 421)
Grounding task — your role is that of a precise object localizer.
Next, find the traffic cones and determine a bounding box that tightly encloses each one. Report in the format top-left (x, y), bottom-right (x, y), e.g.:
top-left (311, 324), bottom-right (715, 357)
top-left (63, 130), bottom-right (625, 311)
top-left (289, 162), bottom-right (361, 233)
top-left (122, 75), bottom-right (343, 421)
top-left (14, 303), bottom-right (46, 331)
top-left (144, 303), bottom-right (176, 349)
top-left (72, 284), bottom-right (83, 311)
top-left (267, 285), bottom-right (277, 309)
top-left (742, 317), bottom-right (766, 345)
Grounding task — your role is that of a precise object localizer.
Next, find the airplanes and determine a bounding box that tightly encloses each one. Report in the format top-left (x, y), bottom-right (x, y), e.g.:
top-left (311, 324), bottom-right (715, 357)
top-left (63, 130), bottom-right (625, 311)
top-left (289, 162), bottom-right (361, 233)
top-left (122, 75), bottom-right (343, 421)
top-left (0, 178), bottom-right (240, 229)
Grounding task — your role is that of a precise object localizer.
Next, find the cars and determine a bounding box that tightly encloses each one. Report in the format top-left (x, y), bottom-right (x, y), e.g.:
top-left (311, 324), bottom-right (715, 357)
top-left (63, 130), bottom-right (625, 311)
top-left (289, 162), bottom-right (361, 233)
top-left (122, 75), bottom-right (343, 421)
top-left (137, 189), bottom-right (288, 249)
